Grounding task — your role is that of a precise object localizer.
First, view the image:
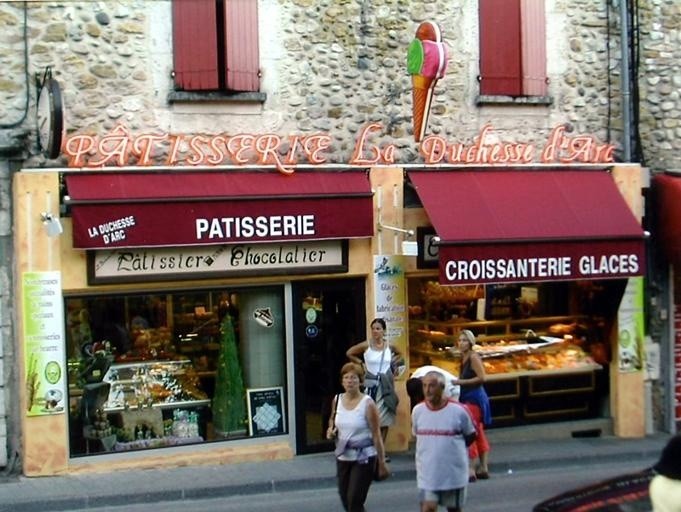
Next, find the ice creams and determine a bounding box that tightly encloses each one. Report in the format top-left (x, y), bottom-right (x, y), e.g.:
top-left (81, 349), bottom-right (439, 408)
top-left (408, 19), bottom-right (448, 142)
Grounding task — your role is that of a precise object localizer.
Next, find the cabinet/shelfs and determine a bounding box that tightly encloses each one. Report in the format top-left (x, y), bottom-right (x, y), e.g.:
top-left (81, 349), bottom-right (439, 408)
top-left (102, 359), bottom-right (210, 440)
top-left (124, 290), bottom-right (212, 331)
top-left (410, 314), bottom-right (604, 429)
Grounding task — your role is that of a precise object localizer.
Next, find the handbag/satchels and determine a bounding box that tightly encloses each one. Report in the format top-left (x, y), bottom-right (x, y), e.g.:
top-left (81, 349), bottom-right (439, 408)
top-left (364, 385), bottom-right (378, 402)
top-left (329, 428), bottom-right (337, 451)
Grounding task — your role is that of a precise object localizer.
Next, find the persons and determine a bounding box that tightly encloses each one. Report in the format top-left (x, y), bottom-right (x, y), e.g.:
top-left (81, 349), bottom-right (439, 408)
top-left (325, 362), bottom-right (389, 511)
top-left (649, 436), bottom-right (681, 512)
top-left (406, 365), bottom-right (460, 403)
top-left (346, 319), bottom-right (405, 463)
top-left (451, 330), bottom-right (492, 482)
top-left (411, 371), bottom-right (479, 512)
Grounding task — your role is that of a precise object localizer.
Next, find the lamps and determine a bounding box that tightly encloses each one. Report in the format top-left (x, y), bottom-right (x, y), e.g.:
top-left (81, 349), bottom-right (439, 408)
top-left (40, 214), bottom-right (63, 236)
top-left (381, 224), bottom-right (418, 256)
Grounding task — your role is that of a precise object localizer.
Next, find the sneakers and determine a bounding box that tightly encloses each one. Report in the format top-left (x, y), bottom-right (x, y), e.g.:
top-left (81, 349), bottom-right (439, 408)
top-left (476, 472), bottom-right (488, 479)
top-left (469, 475), bottom-right (477, 482)
